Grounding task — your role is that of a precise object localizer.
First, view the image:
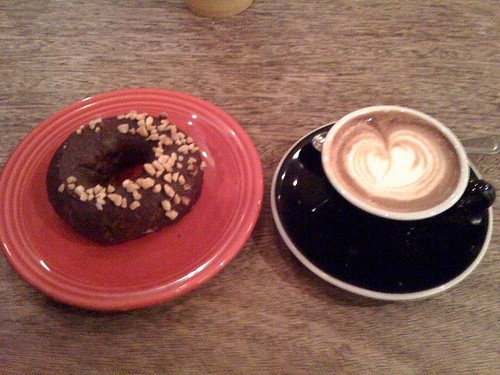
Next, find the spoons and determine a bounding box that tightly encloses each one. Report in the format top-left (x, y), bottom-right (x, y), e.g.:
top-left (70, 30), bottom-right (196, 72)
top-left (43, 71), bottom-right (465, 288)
top-left (310, 130), bottom-right (500, 156)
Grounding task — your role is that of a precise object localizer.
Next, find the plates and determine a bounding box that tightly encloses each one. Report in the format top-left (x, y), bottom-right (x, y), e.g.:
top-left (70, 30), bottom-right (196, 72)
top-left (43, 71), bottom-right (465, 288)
top-left (270, 119), bottom-right (493, 302)
top-left (0, 88), bottom-right (265, 313)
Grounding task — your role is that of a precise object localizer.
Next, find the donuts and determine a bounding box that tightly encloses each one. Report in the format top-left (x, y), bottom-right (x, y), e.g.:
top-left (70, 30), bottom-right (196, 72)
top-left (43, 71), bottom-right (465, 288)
top-left (47, 109), bottom-right (207, 245)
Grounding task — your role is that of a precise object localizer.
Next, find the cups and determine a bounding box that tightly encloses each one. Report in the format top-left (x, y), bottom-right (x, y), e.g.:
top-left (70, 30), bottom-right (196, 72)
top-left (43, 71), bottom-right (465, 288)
top-left (320, 106), bottom-right (495, 221)
top-left (185, 1), bottom-right (253, 18)
